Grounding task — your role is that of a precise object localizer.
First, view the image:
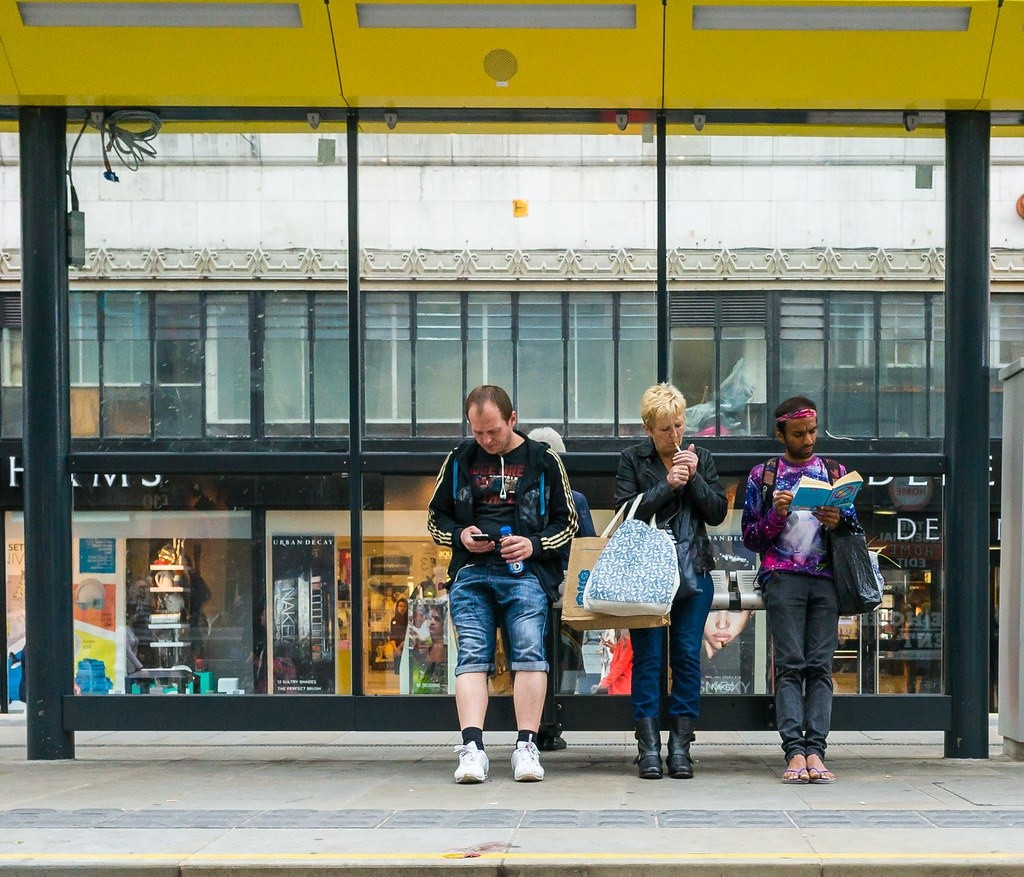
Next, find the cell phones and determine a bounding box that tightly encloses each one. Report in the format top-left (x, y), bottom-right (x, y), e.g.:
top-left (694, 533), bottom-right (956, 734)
top-left (471, 534), bottom-right (492, 543)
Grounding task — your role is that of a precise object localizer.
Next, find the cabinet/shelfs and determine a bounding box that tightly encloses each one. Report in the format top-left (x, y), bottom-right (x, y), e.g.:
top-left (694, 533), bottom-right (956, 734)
top-left (148, 565), bottom-right (192, 647)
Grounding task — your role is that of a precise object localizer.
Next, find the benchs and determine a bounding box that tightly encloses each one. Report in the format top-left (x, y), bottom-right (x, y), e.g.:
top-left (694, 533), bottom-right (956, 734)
top-left (552, 570), bottom-right (767, 609)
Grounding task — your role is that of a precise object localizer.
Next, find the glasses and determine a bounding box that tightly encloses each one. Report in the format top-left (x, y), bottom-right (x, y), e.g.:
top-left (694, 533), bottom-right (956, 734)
top-left (76, 599), bottom-right (105, 611)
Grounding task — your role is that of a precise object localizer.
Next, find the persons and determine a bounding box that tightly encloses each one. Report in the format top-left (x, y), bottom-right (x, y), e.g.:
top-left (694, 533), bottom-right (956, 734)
top-left (427, 384), bottom-right (579, 782)
top-left (381, 586), bottom-right (406, 621)
top-left (612, 383), bottom-right (728, 779)
top-left (740, 395), bottom-right (859, 784)
top-left (390, 597), bottom-right (408, 675)
top-left (500, 427), bottom-right (596, 750)
top-left (409, 604), bottom-right (446, 665)
top-left (592, 627), bottom-right (632, 695)
top-left (702, 608), bottom-right (753, 692)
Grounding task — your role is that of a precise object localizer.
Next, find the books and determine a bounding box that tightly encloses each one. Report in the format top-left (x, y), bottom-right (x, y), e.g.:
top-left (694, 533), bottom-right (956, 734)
top-left (787, 470), bottom-right (863, 512)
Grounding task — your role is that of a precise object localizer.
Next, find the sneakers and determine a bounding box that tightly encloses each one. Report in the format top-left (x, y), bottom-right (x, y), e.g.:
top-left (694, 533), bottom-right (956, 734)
top-left (511, 734), bottom-right (544, 781)
top-left (453, 741), bottom-right (489, 783)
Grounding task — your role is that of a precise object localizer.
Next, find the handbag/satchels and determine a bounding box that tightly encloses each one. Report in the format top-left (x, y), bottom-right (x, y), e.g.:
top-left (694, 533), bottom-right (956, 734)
top-left (561, 500), bottom-right (671, 631)
top-left (583, 493), bottom-right (680, 616)
top-left (829, 514), bottom-right (884, 615)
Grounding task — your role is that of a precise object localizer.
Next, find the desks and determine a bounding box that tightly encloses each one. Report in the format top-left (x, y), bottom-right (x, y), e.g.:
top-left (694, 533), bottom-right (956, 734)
top-left (125, 671), bottom-right (189, 694)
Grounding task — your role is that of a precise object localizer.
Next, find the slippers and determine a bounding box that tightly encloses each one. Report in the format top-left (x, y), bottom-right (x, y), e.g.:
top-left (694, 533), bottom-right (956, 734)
top-left (781, 768), bottom-right (809, 783)
top-left (809, 767), bottom-right (835, 784)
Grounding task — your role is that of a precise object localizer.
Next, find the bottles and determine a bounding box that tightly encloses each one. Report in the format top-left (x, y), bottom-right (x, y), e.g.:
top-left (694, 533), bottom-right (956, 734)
top-left (500, 526), bottom-right (525, 577)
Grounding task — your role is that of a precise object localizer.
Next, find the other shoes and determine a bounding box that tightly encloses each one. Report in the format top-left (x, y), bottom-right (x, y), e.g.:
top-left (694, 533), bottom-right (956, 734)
top-left (516, 734), bottom-right (567, 750)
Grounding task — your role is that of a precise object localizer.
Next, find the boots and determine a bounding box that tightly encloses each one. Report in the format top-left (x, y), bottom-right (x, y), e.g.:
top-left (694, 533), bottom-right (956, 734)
top-left (666, 717), bottom-right (696, 779)
top-left (633, 717), bottom-right (663, 778)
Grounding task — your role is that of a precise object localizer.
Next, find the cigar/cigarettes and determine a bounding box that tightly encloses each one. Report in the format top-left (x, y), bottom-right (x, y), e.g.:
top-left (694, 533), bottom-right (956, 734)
top-left (674, 443), bottom-right (681, 452)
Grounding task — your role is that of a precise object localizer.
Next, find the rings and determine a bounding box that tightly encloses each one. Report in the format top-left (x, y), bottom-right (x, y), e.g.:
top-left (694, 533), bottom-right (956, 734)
top-left (834, 519), bottom-right (837, 523)
